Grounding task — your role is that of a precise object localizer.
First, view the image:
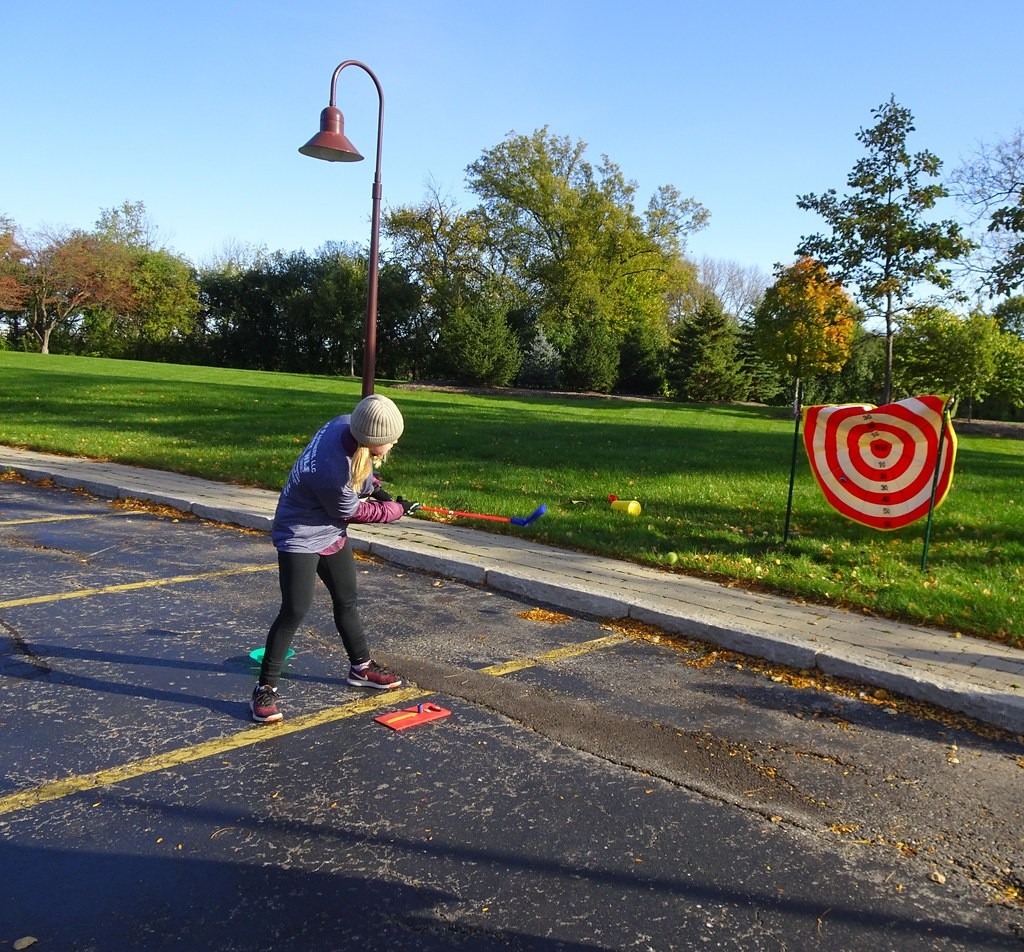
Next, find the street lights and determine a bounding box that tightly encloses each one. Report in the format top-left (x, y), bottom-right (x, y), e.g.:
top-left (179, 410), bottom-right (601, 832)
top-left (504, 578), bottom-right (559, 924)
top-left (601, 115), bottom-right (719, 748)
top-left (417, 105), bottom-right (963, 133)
top-left (300, 59), bottom-right (384, 398)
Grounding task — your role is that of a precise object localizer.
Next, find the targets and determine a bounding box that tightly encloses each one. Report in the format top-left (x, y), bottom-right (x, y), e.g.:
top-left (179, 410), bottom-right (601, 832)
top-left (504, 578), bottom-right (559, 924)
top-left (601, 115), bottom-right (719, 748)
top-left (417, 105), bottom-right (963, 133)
top-left (799, 391), bottom-right (958, 533)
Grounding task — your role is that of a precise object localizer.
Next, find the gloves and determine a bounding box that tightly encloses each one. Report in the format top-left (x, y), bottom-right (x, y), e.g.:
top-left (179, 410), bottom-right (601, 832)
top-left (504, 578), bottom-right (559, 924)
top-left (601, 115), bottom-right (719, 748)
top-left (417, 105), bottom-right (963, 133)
top-left (399, 501), bottom-right (419, 516)
top-left (371, 489), bottom-right (394, 502)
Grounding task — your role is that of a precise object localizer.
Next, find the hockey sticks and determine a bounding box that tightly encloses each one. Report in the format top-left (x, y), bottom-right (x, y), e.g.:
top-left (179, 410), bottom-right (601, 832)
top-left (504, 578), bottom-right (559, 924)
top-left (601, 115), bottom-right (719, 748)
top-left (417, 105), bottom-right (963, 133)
top-left (410, 498), bottom-right (552, 531)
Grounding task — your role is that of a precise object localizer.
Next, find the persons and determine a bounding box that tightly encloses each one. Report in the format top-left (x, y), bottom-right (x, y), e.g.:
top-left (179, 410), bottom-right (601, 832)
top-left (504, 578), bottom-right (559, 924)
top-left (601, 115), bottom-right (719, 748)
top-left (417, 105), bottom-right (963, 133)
top-left (250, 394), bottom-right (423, 723)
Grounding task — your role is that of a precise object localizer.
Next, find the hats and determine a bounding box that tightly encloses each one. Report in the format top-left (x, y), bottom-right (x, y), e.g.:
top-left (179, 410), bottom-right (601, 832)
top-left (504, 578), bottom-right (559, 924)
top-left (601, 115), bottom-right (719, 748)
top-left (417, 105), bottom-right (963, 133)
top-left (350, 394), bottom-right (404, 447)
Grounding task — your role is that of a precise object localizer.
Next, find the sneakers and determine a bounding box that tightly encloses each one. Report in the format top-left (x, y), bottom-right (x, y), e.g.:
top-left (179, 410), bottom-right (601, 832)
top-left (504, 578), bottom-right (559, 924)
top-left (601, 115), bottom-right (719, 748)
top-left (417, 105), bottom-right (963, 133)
top-left (249, 686), bottom-right (284, 722)
top-left (349, 660), bottom-right (401, 689)
